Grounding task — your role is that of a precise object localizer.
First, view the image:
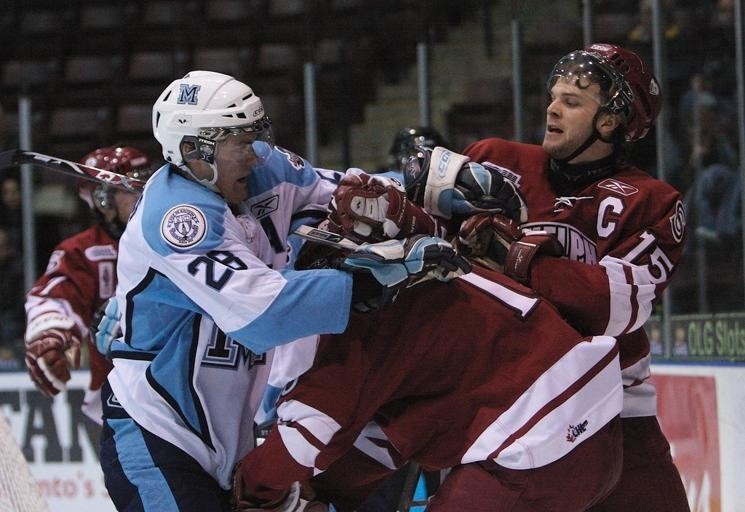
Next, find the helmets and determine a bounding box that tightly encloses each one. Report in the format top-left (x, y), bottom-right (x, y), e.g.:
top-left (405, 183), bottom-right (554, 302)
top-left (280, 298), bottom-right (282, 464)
top-left (393, 126), bottom-right (443, 165)
top-left (77, 145), bottom-right (151, 209)
top-left (152, 70), bottom-right (275, 192)
top-left (547, 44), bottom-right (660, 142)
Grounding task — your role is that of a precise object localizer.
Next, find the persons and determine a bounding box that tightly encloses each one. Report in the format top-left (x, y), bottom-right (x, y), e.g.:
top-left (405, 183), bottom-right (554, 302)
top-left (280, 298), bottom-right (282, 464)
top-left (299, 3), bottom-right (743, 295)
top-left (23, 42), bottom-right (691, 511)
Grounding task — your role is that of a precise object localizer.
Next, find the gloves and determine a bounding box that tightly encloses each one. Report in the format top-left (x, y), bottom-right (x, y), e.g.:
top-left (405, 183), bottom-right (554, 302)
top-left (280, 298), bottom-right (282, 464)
top-left (23, 311), bottom-right (81, 396)
top-left (327, 146), bottom-right (564, 309)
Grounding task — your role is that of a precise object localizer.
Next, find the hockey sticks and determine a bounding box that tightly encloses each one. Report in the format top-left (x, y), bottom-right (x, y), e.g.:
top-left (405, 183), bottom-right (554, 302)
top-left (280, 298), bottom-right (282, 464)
top-left (1, 145), bottom-right (385, 267)
